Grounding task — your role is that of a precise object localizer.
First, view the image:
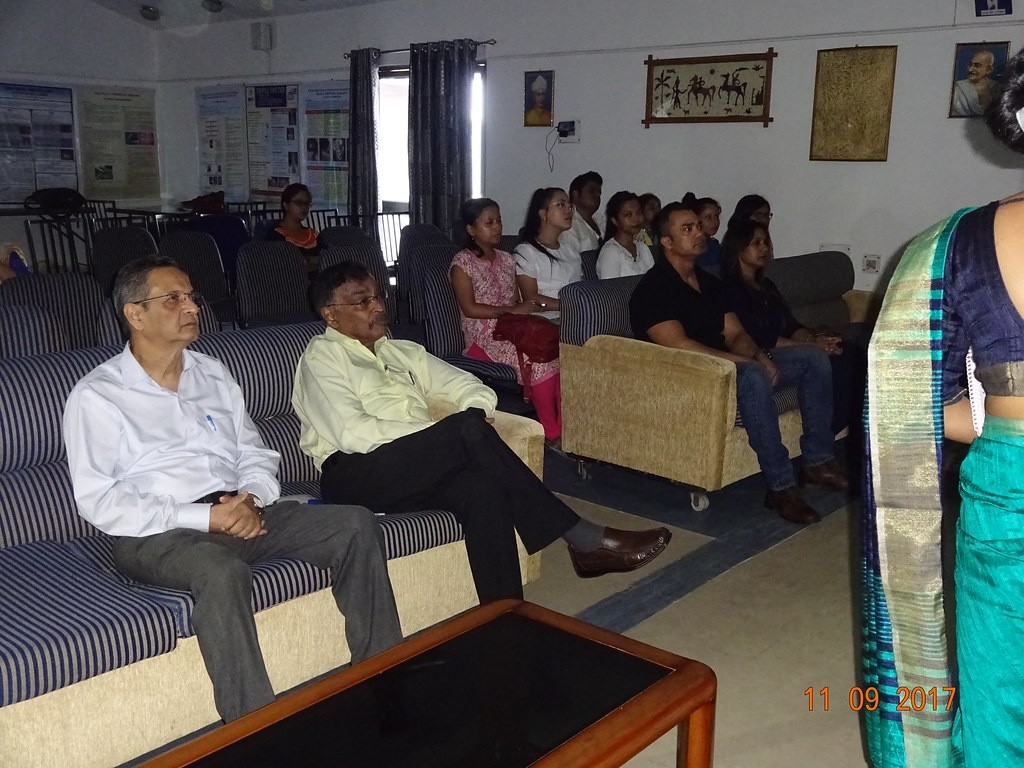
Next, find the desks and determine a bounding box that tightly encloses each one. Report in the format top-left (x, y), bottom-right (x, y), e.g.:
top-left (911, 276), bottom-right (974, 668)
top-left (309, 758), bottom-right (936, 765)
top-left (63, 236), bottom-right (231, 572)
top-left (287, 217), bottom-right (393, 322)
top-left (109, 597), bottom-right (721, 768)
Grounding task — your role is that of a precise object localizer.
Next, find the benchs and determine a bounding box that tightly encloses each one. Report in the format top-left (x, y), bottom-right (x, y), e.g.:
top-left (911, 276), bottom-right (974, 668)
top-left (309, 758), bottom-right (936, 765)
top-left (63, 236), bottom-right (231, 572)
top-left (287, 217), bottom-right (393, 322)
top-left (0, 323), bottom-right (545, 768)
top-left (559, 251), bottom-right (889, 511)
top-left (422, 246), bottom-right (745, 393)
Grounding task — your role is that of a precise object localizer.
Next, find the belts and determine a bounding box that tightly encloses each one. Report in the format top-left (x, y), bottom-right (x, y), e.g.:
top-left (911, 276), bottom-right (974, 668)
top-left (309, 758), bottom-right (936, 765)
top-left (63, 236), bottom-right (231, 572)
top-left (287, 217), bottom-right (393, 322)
top-left (191, 490), bottom-right (239, 505)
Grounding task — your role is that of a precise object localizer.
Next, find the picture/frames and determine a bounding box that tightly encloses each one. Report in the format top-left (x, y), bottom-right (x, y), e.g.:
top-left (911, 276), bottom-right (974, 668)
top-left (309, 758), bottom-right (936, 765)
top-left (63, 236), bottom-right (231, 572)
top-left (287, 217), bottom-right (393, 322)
top-left (524, 70), bottom-right (553, 128)
top-left (808, 44), bottom-right (899, 162)
top-left (947, 41), bottom-right (1012, 117)
top-left (642, 47), bottom-right (779, 129)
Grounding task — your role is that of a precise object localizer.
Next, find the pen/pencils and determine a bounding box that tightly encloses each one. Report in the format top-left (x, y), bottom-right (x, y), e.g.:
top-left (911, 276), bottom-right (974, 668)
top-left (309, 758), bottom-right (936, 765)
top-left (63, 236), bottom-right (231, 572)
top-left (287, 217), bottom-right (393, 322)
top-left (207, 415), bottom-right (216, 431)
top-left (514, 300), bottom-right (548, 307)
top-left (408, 371), bottom-right (415, 384)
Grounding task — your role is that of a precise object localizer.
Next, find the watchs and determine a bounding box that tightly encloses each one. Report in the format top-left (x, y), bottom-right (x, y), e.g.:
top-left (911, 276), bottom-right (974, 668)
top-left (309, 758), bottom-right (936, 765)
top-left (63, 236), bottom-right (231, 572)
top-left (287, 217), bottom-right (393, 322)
top-left (246, 495), bottom-right (265, 515)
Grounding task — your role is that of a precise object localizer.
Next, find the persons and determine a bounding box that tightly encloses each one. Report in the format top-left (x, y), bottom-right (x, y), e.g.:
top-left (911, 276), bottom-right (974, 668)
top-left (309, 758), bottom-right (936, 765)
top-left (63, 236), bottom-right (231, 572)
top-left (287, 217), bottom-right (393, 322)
top-left (0, 241), bottom-right (29, 287)
top-left (857, 46), bottom-right (1024, 768)
top-left (265, 183), bottom-right (324, 278)
top-left (292, 261), bottom-right (672, 606)
top-left (630, 201), bottom-right (862, 524)
top-left (63, 254), bottom-right (403, 725)
top-left (512, 171), bottom-right (773, 323)
top-left (717, 220), bottom-right (868, 430)
top-left (446, 198), bottom-right (576, 461)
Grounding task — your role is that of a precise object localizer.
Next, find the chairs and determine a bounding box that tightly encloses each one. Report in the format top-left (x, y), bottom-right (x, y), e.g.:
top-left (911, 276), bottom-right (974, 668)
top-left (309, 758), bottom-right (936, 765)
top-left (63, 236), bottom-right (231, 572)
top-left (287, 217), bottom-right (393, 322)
top-left (0, 202), bottom-right (521, 359)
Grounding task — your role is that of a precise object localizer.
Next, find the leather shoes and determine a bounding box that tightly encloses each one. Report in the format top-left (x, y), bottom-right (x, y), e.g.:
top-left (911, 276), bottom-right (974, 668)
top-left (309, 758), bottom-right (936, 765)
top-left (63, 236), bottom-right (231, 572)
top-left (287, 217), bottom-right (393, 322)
top-left (777, 499), bottom-right (821, 525)
top-left (568, 527), bottom-right (672, 578)
top-left (801, 462), bottom-right (849, 488)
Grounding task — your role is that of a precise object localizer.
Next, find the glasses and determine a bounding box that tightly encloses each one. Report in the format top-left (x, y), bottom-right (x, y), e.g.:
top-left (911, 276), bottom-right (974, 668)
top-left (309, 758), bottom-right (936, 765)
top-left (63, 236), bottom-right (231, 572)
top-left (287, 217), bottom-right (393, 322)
top-left (753, 212), bottom-right (773, 222)
top-left (549, 200), bottom-right (576, 215)
top-left (326, 290), bottom-right (389, 310)
top-left (290, 199), bottom-right (312, 207)
top-left (134, 291), bottom-right (198, 307)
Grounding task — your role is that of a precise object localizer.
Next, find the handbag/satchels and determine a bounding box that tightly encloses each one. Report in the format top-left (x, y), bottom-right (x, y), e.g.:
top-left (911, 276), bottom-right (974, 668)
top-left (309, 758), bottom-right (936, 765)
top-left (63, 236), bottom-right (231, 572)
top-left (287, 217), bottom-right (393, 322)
top-left (492, 312), bottom-right (560, 404)
